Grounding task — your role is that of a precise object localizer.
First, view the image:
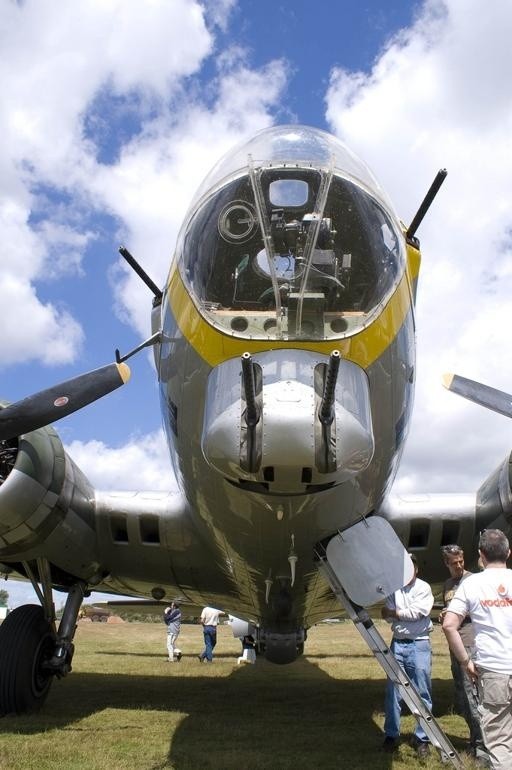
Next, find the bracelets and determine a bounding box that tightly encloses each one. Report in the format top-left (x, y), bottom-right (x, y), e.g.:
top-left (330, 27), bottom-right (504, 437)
top-left (458, 658), bottom-right (471, 665)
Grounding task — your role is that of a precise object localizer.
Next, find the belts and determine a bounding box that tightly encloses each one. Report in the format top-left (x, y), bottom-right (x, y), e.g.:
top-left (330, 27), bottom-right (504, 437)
top-left (393, 638), bottom-right (413, 644)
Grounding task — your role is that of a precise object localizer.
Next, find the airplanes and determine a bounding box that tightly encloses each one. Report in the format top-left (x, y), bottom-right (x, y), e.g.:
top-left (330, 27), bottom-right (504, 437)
top-left (0, 122), bottom-right (512, 723)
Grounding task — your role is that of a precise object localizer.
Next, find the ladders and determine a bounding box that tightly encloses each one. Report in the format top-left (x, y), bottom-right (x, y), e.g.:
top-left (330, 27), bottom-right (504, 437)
top-left (313, 540), bottom-right (467, 770)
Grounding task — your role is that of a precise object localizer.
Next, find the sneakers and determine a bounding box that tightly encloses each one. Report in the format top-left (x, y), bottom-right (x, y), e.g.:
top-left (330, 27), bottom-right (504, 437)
top-left (199, 655), bottom-right (203, 662)
top-left (416, 739), bottom-right (429, 758)
top-left (176, 652), bottom-right (183, 662)
top-left (383, 735), bottom-right (400, 752)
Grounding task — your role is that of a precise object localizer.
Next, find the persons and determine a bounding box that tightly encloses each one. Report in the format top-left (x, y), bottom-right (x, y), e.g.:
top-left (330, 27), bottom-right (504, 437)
top-left (441, 526), bottom-right (512, 770)
top-left (381, 551), bottom-right (436, 759)
top-left (438, 543), bottom-right (490, 761)
top-left (162, 602), bottom-right (183, 663)
top-left (196, 605), bottom-right (226, 664)
top-left (237, 634), bottom-right (258, 666)
